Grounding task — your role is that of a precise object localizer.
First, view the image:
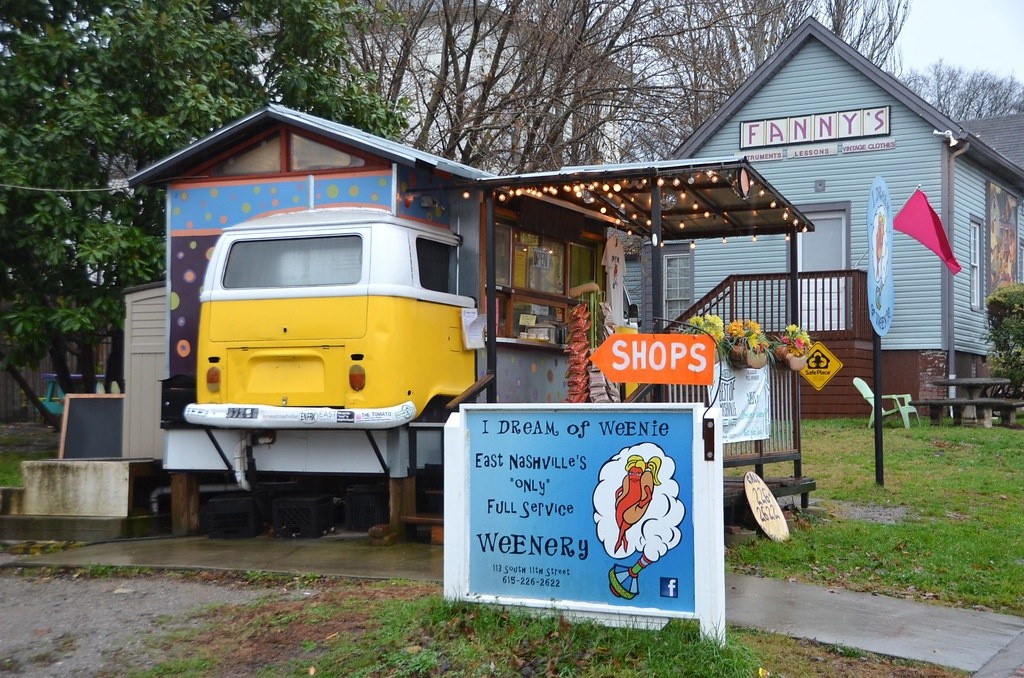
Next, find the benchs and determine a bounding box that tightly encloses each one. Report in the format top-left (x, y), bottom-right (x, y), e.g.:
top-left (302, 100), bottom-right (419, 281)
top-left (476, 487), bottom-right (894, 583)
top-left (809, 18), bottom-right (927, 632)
top-left (908, 400), bottom-right (1024, 428)
top-left (37, 397), bottom-right (60, 404)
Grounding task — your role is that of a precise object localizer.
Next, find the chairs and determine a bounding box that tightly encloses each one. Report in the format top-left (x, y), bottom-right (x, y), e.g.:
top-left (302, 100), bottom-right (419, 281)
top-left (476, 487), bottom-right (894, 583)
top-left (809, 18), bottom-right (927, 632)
top-left (852, 378), bottom-right (921, 428)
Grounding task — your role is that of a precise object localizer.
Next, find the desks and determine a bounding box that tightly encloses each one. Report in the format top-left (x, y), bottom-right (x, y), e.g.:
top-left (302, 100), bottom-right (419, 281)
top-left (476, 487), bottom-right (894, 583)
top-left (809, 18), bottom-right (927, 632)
top-left (934, 378), bottom-right (1012, 424)
top-left (45, 373), bottom-right (105, 414)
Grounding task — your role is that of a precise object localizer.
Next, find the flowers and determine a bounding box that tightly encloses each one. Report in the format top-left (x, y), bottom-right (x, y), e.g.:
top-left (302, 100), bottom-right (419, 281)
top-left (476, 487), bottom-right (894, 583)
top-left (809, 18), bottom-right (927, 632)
top-left (685, 315), bottom-right (727, 362)
top-left (724, 320), bottom-right (779, 368)
top-left (774, 323), bottom-right (814, 359)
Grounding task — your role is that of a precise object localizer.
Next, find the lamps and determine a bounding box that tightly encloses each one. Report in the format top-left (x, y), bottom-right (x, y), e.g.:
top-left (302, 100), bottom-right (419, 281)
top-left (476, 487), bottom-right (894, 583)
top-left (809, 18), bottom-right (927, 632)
top-left (933, 129), bottom-right (958, 147)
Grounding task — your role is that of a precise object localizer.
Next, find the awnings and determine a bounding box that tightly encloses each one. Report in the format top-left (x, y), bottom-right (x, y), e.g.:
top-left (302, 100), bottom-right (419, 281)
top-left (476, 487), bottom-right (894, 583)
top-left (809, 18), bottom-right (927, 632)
top-left (479, 156), bottom-right (815, 405)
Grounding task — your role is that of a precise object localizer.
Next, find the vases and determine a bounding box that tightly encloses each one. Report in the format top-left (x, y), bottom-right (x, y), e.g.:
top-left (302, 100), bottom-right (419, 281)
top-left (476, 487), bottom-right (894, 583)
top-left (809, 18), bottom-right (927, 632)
top-left (775, 345), bottom-right (807, 370)
top-left (729, 346), bottom-right (767, 369)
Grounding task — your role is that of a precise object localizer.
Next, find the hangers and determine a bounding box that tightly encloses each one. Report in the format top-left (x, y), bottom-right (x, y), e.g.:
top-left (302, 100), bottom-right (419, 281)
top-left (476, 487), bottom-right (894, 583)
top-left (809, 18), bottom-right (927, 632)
top-left (611, 223), bottom-right (619, 241)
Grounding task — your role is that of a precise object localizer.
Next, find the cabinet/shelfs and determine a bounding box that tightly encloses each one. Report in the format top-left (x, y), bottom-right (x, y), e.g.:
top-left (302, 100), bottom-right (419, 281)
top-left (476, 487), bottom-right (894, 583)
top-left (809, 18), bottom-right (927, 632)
top-left (581, 290), bottom-right (607, 351)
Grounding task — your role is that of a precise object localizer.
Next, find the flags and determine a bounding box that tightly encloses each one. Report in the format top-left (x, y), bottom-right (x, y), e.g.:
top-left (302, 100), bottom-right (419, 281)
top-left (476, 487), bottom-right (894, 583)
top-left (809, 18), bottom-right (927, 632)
top-left (892, 188), bottom-right (962, 275)
